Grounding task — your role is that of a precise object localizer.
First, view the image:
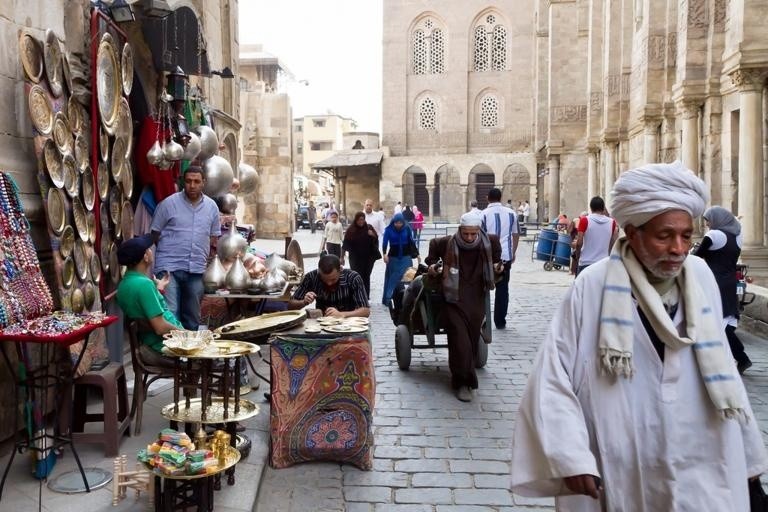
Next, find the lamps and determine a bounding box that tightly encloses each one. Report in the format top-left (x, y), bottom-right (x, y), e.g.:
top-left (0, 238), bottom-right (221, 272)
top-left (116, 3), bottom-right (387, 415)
top-left (208, 61), bottom-right (234, 80)
top-left (95, 0), bottom-right (136, 23)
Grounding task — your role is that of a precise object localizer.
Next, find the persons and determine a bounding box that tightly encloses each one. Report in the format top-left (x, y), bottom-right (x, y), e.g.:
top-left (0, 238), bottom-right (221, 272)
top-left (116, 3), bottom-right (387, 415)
top-left (116, 233), bottom-right (252, 399)
top-left (479, 188), bottom-right (521, 328)
top-left (535, 198), bottom-right (538, 221)
top-left (148, 164), bottom-right (222, 330)
top-left (694, 205), bottom-right (752, 375)
top-left (551, 196), bottom-right (620, 278)
top-left (460, 200), bottom-right (483, 216)
top-left (506, 199), bottom-right (529, 231)
top-left (507, 159), bottom-right (768, 512)
top-left (286, 186), bottom-right (424, 319)
top-left (424, 211), bottom-right (505, 402)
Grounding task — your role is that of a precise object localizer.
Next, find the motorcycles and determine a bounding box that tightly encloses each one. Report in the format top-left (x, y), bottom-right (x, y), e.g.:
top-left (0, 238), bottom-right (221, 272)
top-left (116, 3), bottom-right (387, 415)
top-left (689, 241), bottom-right (757, 316)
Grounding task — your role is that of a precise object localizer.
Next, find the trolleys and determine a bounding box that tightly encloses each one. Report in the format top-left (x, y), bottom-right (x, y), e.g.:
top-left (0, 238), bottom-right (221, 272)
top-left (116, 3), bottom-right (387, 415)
top-left (532, 233), bottom-right (575, 272)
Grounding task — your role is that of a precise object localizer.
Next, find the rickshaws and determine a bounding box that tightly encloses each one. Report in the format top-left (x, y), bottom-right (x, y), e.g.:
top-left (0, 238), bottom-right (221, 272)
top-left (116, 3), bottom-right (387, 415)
top-left (394, 256), bottom-right (508, 372)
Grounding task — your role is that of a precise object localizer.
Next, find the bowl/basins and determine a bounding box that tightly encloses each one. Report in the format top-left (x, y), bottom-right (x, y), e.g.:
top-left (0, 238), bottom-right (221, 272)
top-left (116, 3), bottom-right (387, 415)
top-left (162, 328), bottom-right (214, 355)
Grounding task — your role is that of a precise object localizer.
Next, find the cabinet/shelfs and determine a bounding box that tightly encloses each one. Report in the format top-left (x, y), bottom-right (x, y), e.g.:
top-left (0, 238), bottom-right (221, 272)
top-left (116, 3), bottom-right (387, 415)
top-left (157, 338), bottom-right (261, 491)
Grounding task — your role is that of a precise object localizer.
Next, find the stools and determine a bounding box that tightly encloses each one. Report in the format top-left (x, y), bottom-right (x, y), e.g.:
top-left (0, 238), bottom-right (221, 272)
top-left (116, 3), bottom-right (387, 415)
top-left (52, 361), bottom-right (133, 458)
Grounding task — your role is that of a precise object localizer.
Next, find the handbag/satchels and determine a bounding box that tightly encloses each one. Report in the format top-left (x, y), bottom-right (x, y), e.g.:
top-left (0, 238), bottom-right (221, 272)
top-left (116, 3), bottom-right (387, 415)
top-left (409, 237), bottom-right (420, 258)
top-left (368, 237), bottom-right (382, 261)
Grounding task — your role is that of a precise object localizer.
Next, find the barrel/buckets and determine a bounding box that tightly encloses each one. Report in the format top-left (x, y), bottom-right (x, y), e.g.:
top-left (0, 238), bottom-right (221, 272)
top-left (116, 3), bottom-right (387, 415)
top-left (555, 234), bottom-right (572, 265)
top-left (536, 229), bottom-right (558, 261)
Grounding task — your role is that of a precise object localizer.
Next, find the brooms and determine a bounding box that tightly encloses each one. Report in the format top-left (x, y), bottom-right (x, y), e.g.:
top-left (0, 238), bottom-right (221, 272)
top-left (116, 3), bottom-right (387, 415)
top-left (22, 342), bottom-right (55, 479)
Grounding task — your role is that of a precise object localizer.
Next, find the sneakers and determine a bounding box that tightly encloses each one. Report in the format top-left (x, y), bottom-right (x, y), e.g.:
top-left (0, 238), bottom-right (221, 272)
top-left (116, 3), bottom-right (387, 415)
top-left (456, 384), bottom-right (472, 403)
top-left (737, 353), bottom-right (752, 375)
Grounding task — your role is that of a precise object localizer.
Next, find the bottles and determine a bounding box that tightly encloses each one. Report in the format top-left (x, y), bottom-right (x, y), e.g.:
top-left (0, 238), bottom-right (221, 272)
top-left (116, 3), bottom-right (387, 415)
top-left (193, 424), bottom-right (232, 465)
top-left (202, 219), bottom-right (294, 294)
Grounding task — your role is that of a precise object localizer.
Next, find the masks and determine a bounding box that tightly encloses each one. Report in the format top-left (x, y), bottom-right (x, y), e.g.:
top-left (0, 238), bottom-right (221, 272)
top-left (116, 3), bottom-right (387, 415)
top-left (116, 232), bottom-right (153, 266)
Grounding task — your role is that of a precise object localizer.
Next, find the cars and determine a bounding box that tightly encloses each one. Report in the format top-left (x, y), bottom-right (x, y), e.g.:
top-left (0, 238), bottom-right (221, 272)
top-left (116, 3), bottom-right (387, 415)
top-left (296, 206), bottom-right (318, 229)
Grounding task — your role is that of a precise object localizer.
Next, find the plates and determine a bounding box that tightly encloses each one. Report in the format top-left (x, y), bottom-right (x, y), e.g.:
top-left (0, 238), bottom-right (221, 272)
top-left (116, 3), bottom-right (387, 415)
top-left (305, 316), bottom-right (369, 333)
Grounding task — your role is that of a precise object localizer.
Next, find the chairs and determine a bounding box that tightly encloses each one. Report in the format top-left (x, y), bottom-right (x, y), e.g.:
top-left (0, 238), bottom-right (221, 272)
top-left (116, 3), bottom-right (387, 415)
top-left (129, 317), bottom-right (213, 437)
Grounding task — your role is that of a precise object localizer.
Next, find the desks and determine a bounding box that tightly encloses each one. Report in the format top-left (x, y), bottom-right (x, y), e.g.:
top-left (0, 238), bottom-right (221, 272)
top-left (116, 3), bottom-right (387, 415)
top-left (0, 313), bottom-right (120, 512)
top-left (268, 315), bottom-right (373, 472)
top-left (205, 281), bottom-right (291, 323)
